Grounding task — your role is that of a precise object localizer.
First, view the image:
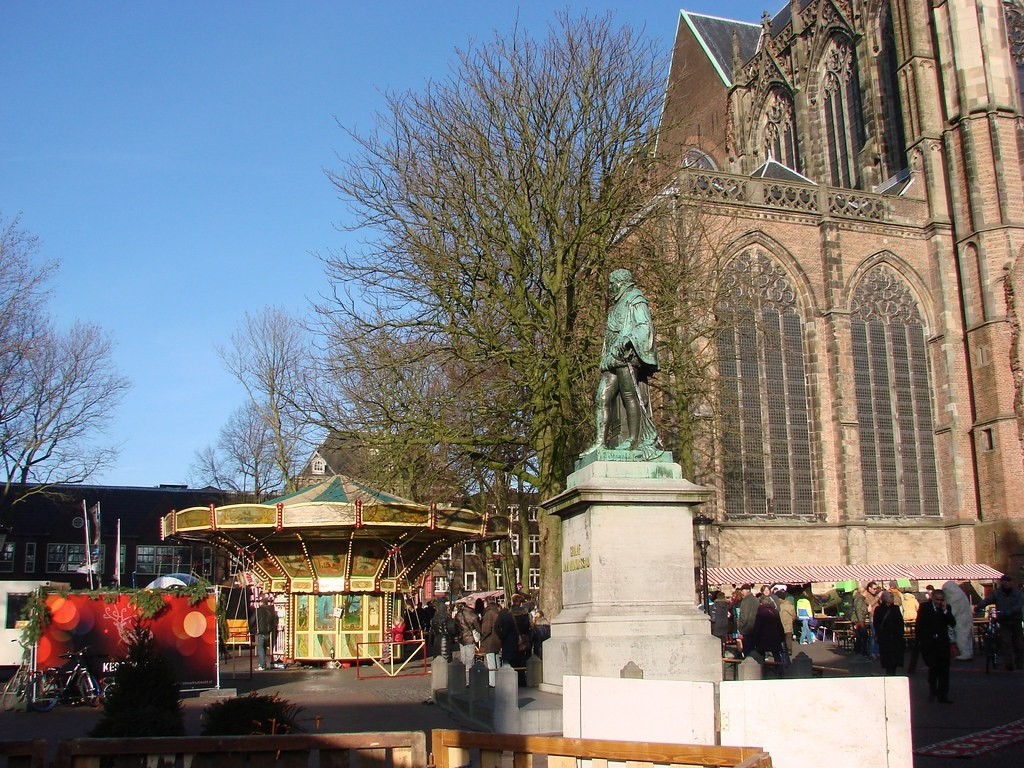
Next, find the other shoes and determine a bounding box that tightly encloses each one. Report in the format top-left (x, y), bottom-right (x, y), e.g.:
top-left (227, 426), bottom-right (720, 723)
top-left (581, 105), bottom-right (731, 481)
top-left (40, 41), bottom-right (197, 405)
top-left (1006, 664), bottom-right (1013, 670)
top-left (937, 698), bottom-right (955, 703)
top-left (254, 666), bottom-right (266, 671)
top-left (955, 655), bottom-right (972, 660)
top-left (800, 641), bottom-right (808, 645)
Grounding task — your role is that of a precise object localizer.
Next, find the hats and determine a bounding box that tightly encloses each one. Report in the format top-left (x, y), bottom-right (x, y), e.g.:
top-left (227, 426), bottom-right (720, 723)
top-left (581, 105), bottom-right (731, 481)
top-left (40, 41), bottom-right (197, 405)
top-left (260, 597), bottom-right (268, 604)
top-left (1000, 576), bottom-right (1012, 582)
top-left (738, 584), bottom-right (750, 590)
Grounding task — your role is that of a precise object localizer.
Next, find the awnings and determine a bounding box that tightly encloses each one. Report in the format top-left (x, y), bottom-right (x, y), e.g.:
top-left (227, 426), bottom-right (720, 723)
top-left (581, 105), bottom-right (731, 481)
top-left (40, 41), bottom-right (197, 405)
top-left (701, 563), bottom-right (1007, 592)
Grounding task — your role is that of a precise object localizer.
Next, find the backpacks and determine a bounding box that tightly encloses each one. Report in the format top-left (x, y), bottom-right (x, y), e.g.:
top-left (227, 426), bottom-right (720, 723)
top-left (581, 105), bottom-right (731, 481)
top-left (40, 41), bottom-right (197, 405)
top-left (471, 628), bottom-right (481, 643)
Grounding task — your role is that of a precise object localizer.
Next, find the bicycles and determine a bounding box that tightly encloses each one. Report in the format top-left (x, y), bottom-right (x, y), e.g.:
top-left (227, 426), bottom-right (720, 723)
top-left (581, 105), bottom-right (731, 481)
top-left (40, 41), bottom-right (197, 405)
top-left (0, 639), bottom-right (33, 712)
top-left (26, 644), bottom-right (102, 714)
top-left (99, 677), bottom-right (116, 702)
top-left (976, 608), bottom-right (1004, 674)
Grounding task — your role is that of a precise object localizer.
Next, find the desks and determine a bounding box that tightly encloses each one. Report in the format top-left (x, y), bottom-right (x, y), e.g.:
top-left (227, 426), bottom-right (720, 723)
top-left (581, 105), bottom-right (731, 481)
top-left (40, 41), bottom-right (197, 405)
top-left (817, 615), bottom-right (989, 657)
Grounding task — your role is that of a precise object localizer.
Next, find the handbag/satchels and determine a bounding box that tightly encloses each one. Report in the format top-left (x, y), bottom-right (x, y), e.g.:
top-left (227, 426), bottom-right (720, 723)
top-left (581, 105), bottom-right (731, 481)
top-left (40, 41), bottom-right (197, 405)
top-left (518, 636), bottom-right (532, 653)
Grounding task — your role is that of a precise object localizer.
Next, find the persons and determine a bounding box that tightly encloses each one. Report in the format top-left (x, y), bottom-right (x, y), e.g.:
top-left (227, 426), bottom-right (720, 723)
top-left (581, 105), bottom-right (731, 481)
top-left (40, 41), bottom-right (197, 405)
top-left (250, 598), bottom-right (278, 671)
top-left (707, 580), bottom-right (1023, 703)
top-left (579, 270), bottom-right (661, 457)
top-left (406, 594), bottom-right (550, 684)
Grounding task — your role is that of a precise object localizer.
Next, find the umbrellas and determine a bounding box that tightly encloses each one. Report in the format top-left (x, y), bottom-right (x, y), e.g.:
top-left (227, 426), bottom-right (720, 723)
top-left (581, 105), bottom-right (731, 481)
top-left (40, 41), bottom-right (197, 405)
top-left (144, 573), bottom-right (198, 589)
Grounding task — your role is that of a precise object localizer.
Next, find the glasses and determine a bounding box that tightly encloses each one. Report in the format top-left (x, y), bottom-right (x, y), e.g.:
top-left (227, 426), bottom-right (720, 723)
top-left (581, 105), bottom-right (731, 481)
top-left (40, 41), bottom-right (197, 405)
top-left (934, 598), bottom-right (944, 602)
top-left (872, 588), bottom-right (877, 590)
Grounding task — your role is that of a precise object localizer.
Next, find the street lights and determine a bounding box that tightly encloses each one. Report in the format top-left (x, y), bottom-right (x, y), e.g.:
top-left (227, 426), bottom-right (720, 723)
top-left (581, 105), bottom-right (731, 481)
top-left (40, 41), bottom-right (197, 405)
top-left (692, 512), bottom-right (714, 616)
top-left (445, 566), bottom-right (457, 618)
